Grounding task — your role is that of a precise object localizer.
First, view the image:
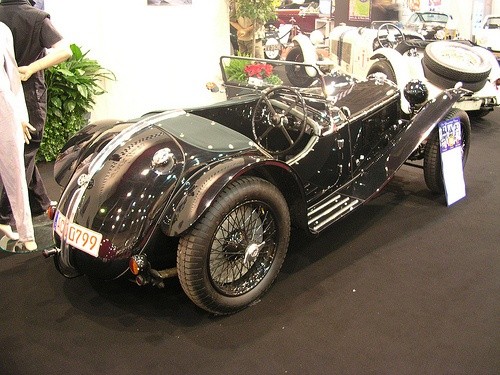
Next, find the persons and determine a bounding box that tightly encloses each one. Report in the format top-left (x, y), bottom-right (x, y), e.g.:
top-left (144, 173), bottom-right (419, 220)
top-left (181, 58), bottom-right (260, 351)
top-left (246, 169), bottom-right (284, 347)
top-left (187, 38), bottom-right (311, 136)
top-left (0, 0), bottom-right (73, 253)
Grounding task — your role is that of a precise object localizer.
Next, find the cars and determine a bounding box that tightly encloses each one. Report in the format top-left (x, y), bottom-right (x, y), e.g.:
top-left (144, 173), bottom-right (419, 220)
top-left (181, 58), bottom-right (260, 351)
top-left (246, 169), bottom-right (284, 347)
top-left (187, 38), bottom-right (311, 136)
top-left (43, 11), bottom-right (500, 319)
